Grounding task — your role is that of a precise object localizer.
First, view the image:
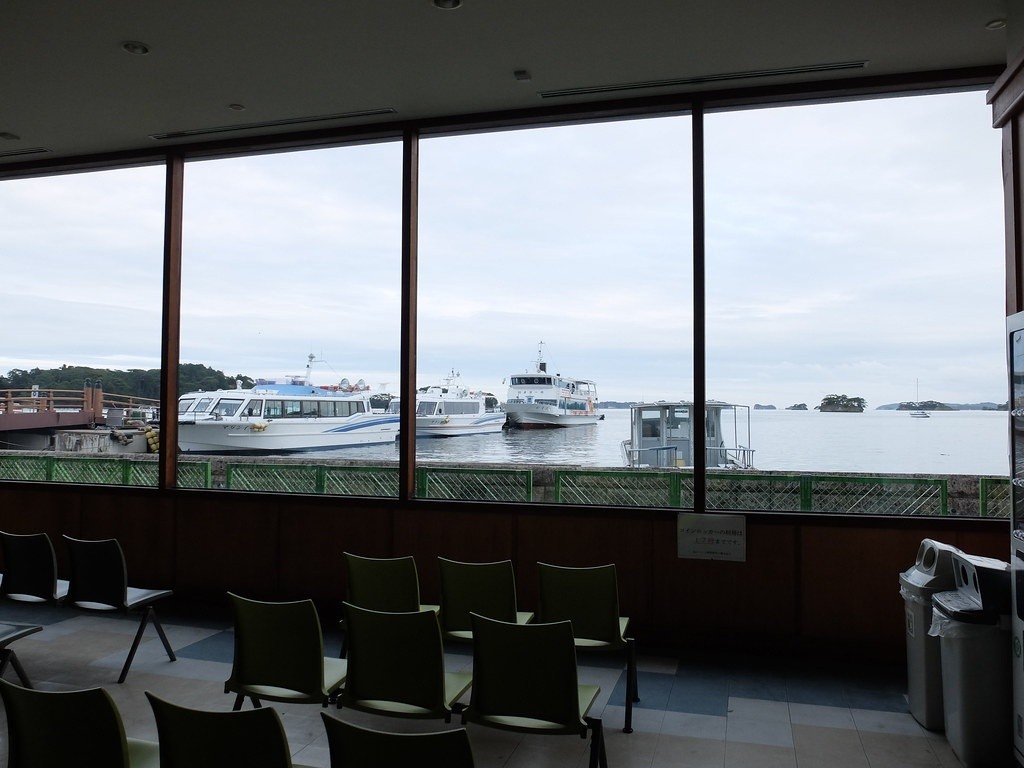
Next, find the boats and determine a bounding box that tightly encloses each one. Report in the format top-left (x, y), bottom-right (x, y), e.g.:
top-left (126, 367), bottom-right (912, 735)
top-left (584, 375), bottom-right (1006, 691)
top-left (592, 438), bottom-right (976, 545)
top-left (384, 366), bottom-right (506, 436)
top-left (147, 353), bottom-right (401, 452)
top-left (621, 401), bottom-right (755, 470)
top-left (502, 341), bottom-right (604, 427)
top-left (910, 409), bottom-right (931, 420)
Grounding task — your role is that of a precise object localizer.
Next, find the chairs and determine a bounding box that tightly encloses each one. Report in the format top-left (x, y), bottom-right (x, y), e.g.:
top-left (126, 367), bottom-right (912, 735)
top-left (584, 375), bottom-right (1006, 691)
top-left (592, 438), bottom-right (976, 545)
top-left (0, 531), bottom-right (177, 689)
top-left (224, 552), bottom-right (640, 768)
top-left (0, 676), bottom-right (474, 768)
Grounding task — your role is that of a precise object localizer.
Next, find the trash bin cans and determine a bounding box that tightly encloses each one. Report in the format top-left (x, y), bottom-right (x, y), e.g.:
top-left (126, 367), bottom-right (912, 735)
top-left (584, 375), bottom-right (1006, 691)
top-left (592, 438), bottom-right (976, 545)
top-left (899, 537), bottom-right (966, 733)
top-left (932, 549), bottom-right (1011, 768)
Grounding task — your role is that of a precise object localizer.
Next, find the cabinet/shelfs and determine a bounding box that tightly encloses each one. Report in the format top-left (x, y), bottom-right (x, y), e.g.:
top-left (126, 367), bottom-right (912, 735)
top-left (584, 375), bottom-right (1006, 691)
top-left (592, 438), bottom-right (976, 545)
top-left (983, 54), bottom-right (1024, 768)
